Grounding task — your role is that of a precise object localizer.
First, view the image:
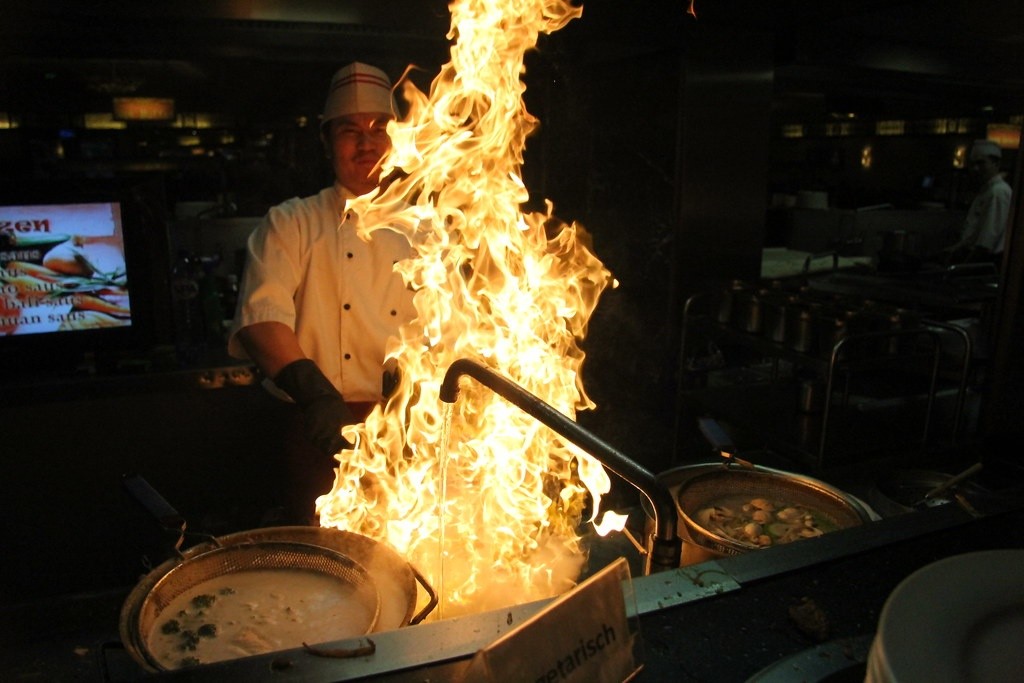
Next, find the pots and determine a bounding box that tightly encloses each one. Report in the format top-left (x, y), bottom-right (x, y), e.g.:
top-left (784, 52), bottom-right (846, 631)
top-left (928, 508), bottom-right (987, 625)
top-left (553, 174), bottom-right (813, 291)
top-left (877, 228), bottom-right (930, 263)
top-left (639, 458), bottom-right (885, 579)
top-left (99, 468), bottom-right (438, 683)
top-left (864, 467), bottom-right (986, 524)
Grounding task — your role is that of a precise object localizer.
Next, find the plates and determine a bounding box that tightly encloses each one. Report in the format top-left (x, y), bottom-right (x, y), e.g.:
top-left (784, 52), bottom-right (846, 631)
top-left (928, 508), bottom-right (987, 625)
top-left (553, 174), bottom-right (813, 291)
top-left (862, 548), bottom-right (1024, 683)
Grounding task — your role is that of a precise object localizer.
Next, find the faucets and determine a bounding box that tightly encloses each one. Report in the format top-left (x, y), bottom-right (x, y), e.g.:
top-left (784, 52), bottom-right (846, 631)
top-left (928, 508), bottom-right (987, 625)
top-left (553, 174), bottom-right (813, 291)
top-left (438, 357), bottom-right (683, 577)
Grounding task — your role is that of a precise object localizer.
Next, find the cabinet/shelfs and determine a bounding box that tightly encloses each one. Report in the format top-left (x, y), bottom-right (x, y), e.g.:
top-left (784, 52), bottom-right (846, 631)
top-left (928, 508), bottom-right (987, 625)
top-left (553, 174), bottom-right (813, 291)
top-left (670, 288), bottom-right (941, 474)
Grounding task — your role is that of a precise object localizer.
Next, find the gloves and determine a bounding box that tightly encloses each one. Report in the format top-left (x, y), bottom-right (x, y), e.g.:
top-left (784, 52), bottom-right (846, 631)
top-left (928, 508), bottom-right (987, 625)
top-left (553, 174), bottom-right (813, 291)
top-left (281, 358), bottom-right (353, 456)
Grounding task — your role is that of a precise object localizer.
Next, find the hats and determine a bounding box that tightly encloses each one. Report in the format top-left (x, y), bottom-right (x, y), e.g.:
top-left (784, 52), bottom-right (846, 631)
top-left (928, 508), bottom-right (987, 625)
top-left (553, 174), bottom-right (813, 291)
top-left (970, 140), bottom-right (1001, 157)
top-left (320, 62), bottom-right (401, 125)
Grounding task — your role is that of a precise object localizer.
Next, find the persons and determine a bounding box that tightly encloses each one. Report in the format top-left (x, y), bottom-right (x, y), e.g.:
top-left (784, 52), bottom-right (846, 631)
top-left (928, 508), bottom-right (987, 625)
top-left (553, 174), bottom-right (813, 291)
top-left (955, 139), bottom-right (1012, 276)
top-left (225, 62), bottom-right (430, 527)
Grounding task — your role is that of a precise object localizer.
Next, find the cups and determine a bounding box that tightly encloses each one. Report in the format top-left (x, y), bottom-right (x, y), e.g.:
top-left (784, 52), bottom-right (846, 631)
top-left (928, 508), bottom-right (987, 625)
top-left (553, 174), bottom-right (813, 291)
top-left (795, 376), bottom-right (824, 414)
top-left (707, 276), bottom-right (922, 364)
